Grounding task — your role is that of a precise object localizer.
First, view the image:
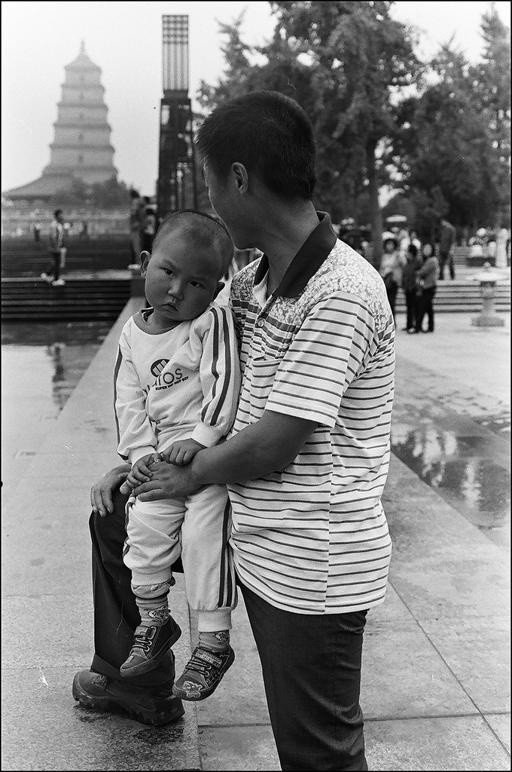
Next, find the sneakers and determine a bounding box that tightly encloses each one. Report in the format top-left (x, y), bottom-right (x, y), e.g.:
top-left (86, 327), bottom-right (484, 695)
top-left (72, 671), bottom-right (185, 727)
top-left (119, 611), bottom-right (181, 678)
top-left (171, 643), bottom-right (236, 703)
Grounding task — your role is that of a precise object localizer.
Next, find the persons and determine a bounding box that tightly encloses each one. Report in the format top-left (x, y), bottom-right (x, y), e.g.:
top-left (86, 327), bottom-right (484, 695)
top-left (112, 209), bottom-right (241, 701)
top-left (72, 91), bottom-right (397, 772)
